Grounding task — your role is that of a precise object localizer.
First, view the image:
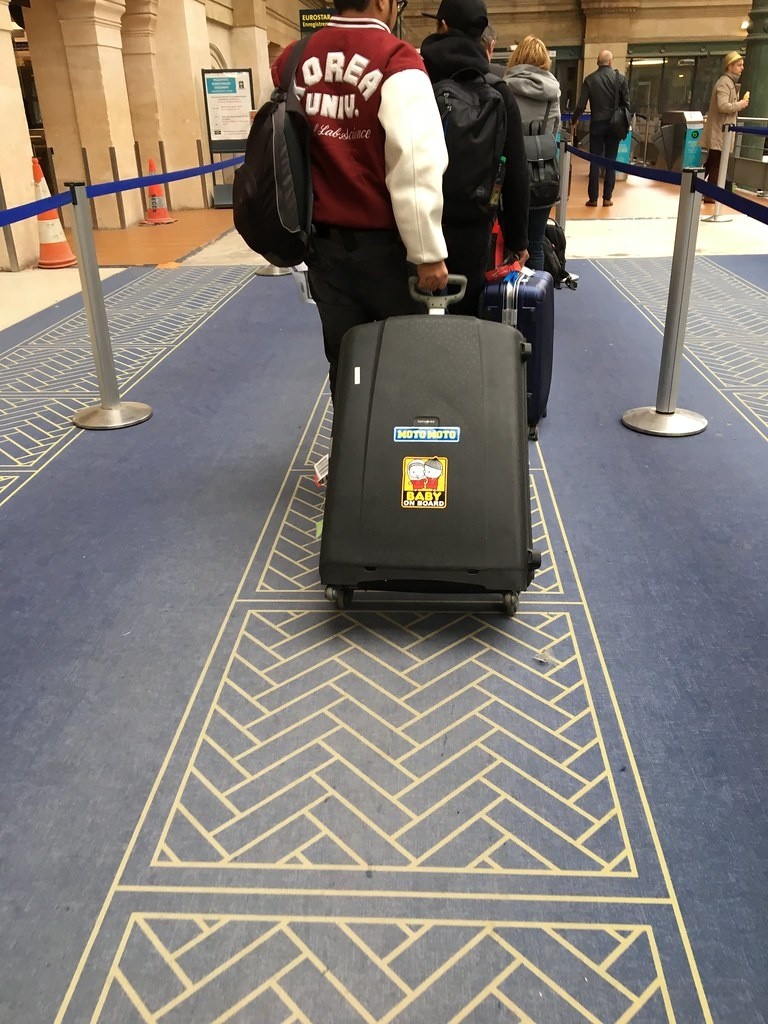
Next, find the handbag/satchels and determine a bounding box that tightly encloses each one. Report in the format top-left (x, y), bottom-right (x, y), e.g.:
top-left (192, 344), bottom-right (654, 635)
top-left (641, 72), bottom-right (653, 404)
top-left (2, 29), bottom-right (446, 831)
top-left (609, 103), bottom-right (631, 144)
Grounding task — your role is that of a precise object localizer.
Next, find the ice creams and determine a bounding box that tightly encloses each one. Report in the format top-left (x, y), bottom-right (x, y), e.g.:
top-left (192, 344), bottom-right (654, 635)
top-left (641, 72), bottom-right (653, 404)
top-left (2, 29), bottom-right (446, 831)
top-left (743, 91), bottom-right (750, 99)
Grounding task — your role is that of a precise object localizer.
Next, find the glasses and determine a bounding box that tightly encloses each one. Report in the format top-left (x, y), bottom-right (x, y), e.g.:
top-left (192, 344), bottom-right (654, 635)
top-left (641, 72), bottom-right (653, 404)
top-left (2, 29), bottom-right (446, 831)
top-left (396, 0), bottom-right (408, 16)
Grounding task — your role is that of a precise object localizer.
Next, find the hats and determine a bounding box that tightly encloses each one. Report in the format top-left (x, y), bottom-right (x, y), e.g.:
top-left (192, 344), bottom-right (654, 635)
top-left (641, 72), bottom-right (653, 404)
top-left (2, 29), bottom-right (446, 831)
top-left (421, 0), bottom-right (488, 37)
top-left (722, 50), bottom-right (747, 72)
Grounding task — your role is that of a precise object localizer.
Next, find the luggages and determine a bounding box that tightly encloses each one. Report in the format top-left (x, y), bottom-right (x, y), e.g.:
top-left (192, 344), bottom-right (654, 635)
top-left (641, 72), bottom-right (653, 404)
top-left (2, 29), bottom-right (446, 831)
top-left (479, 253), bottom-right (555, 442)
top-left (320, 275), bottom-right (538, 620)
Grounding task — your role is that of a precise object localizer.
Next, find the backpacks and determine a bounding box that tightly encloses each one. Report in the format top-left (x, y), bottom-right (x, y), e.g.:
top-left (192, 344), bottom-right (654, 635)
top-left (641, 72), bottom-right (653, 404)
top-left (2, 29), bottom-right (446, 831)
top-left (545, 216), bottom-right (566, 271)
top-left (418, 76), bottom-right (508, 229)
top-left (231, 36), bottom-right (315, 268)
top-left (515, 118), bottom-right (563, 210)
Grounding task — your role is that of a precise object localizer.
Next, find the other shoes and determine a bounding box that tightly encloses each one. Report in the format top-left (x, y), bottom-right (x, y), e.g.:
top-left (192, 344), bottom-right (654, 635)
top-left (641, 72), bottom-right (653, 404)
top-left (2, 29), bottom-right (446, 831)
top-left (603, 199), bottom-right (613, 206)
top-left (558, 271), bottom-right (578, 283)
top-left (586, 198), bottom-right (597, 207)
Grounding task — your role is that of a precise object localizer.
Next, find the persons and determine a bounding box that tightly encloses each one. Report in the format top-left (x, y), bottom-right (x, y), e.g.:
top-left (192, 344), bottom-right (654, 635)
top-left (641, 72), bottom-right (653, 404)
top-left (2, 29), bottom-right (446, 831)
top-left (570, 50), bottom-right (630, 206)
top-left (698, 50), bottom-right (748, 202)
top-left (272, 0), bottom-right (579, 436)
